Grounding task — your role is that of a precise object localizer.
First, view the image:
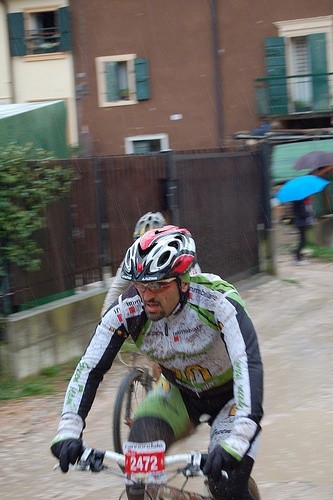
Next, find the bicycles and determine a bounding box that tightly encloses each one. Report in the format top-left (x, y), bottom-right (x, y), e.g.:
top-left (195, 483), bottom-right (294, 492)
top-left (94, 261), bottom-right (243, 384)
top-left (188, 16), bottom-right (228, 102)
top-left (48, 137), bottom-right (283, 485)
top-left (52, 414), bottom-right (263, 500)
top-left (112, 347), bottom-right (161, 474)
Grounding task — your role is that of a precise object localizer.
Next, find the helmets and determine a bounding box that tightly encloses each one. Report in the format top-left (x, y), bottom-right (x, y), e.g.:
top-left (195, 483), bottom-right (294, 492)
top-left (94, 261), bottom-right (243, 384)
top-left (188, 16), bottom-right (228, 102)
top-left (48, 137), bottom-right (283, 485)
top-left (120, 226), bottom-right (195, 281)
top-left (132, 210), bottom-right (165, 239)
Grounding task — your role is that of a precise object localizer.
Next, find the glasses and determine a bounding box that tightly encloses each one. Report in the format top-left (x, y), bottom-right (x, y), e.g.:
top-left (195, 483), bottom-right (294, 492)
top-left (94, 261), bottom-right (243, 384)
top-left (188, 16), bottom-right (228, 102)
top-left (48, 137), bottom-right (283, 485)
top-left (132, 282), bottom-right (171, 292)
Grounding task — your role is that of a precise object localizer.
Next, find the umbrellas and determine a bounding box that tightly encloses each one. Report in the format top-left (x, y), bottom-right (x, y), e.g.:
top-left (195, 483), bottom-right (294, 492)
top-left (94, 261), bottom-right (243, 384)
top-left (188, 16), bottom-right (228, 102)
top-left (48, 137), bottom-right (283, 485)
top-left (293, 147), bottom-right (332, 171)
top-left (272, 173), bottom-right (330, 206)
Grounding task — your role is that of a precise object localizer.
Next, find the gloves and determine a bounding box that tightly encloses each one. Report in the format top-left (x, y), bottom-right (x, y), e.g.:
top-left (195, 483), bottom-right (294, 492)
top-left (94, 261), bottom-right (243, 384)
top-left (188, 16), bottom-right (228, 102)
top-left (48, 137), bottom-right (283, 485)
top-left (202, 417), bottom-right (260, 479)
top-left (51, 413), bottom-right (86, 473)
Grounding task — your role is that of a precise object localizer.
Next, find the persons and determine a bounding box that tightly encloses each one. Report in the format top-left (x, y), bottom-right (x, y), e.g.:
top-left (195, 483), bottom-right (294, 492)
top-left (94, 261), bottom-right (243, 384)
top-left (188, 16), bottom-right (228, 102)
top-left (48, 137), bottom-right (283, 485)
top-left (50, 225), bottom-right (264, 500)
top-left (100, 212), bottom-right (168, 319)
top-left (289, 192), bottom-right (317, 262)
